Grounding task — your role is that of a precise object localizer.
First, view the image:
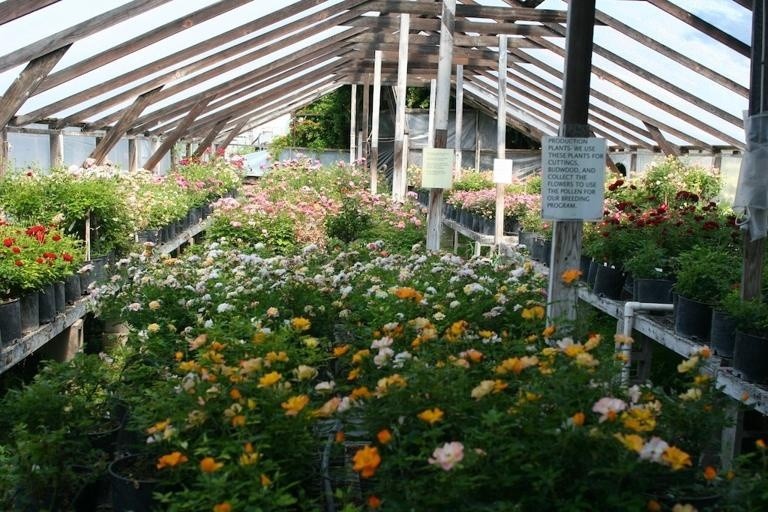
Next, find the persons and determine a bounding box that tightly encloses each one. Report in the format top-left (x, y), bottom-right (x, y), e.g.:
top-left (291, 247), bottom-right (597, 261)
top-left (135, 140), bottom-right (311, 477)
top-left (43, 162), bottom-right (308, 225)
top-left (615, 161), bottom-right (626, 178)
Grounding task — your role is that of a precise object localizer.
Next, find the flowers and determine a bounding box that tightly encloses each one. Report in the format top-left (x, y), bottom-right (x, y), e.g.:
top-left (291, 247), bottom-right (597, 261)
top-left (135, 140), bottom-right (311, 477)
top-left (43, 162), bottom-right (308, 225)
top-left (0, 151), bottom-right (763, 511)
